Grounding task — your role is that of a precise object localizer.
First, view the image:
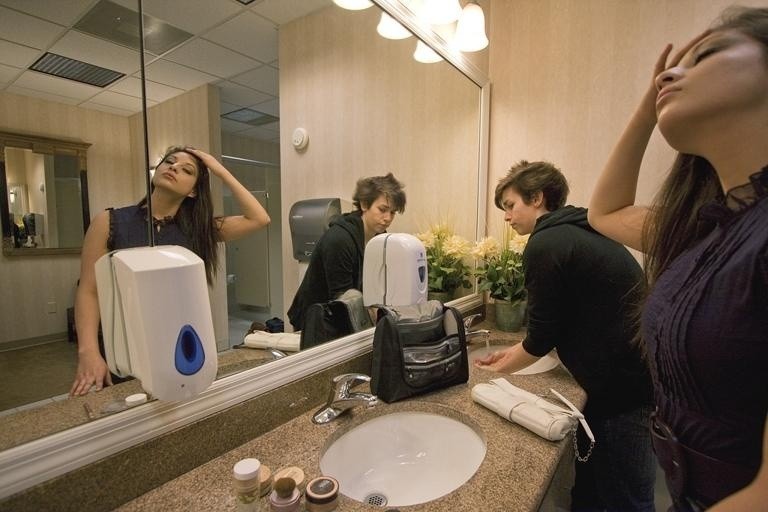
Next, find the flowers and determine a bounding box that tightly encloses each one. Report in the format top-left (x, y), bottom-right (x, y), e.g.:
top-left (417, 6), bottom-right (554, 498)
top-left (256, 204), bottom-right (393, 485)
top-left (472, 222), bottom-right (528, 303)
top-left (412, 214), bottom-right (472, 292)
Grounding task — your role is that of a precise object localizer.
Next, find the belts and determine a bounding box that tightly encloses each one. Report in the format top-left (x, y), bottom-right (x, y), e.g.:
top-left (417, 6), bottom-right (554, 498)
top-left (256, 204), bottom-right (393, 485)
top-left (645, 415), bottom-right (761, 504)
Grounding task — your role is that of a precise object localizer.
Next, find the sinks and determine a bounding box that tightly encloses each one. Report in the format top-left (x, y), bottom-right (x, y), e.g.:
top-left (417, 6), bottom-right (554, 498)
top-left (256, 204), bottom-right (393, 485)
top-left (313, 398), bottom-right (490, 512)
top-left (214, 355), bottom-right (275, 381)
top-left (459, 332), bottom-right (561, 378)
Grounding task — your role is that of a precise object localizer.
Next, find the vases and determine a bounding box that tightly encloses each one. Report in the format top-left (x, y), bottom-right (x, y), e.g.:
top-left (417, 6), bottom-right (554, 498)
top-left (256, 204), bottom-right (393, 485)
top-left (427, 290), bottom-right (450, 302)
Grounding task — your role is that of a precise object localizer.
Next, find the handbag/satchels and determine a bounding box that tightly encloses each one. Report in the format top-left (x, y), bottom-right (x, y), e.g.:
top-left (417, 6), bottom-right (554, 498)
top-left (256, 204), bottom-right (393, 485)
top-left (471, 376), bottom-right (596, 464)
top-left (233, 330), bottom-right (301, 352)
top-left (369, 300), bottom-right (471, 405)
top-left (300, 289), bottom-right (373, 353)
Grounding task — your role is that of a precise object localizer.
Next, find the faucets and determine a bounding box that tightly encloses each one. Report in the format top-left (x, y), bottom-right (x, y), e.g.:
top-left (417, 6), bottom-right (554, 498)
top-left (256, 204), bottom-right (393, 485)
top-left (263, 344), bottom-right (288, 359)
top-left (461, 309), bottom-right (492, 344)
top-left (310, 371), bottom-right (378, 426)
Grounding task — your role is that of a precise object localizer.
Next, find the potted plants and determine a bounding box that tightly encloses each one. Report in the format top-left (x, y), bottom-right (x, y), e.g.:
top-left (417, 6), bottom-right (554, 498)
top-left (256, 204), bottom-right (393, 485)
top-left (491, 298), bottom-right (527, 332)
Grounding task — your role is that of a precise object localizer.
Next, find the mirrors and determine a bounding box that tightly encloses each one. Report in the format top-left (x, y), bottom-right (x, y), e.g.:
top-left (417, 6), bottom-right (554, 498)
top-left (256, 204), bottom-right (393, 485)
top-left (1, 131), bottom-right (94, 257)
top-left (1, 1), bottom-right (491, 454)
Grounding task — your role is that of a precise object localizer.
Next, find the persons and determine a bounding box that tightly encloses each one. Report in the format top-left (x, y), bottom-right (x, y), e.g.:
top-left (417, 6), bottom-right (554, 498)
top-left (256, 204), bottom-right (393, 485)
top-left (474, 159), bottom-right (656, 512)
top-left (587, 7), bottom-right (768, 512)
top-left (70, 147), bottom-right (272, 398)
top-left (287, 173), bottom-right (407, 332)
